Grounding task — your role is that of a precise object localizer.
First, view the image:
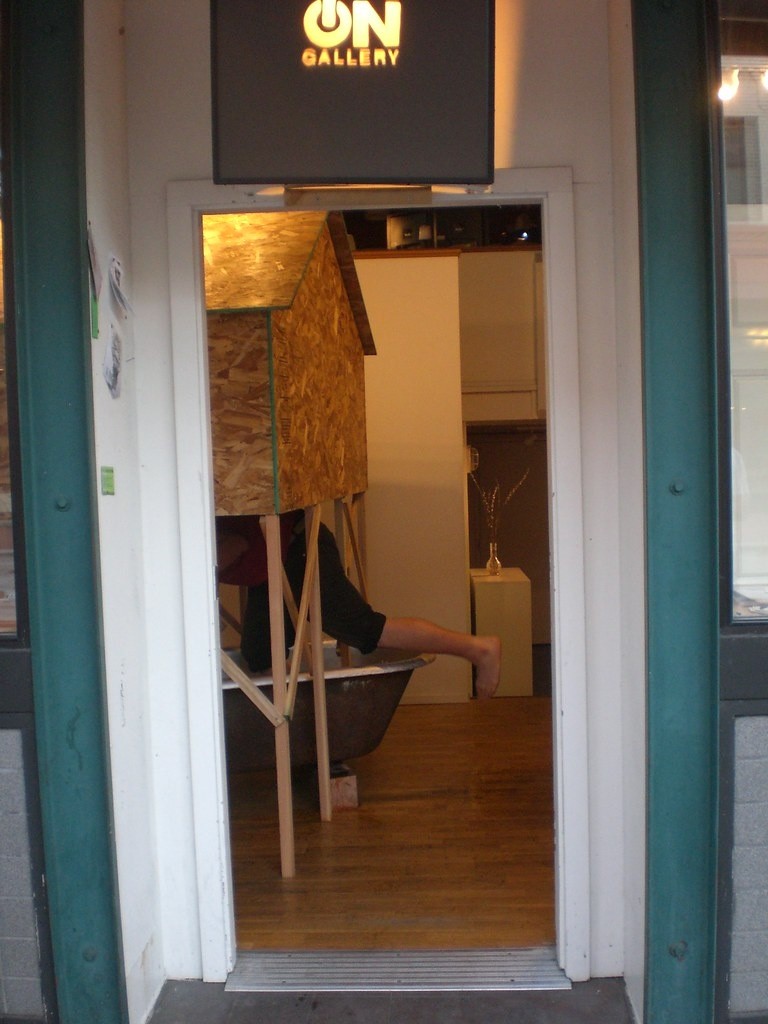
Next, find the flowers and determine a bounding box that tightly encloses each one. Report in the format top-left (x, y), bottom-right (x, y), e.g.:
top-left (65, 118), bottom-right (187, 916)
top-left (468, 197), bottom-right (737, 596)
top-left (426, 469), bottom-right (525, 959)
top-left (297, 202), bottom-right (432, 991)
top-left (470, 466), bottom-right (531, 543)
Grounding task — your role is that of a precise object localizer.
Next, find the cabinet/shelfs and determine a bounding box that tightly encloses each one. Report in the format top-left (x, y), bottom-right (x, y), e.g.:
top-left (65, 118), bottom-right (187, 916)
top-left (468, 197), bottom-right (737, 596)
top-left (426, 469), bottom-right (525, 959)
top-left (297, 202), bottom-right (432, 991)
top-left (469, 567), bottom-right (533, 698)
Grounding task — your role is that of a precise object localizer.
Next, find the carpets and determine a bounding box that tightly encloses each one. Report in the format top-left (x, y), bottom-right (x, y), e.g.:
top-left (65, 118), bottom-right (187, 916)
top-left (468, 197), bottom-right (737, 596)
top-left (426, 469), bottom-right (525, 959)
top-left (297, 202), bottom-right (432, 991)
top-left (145, 975), bottom-right (637, 1023)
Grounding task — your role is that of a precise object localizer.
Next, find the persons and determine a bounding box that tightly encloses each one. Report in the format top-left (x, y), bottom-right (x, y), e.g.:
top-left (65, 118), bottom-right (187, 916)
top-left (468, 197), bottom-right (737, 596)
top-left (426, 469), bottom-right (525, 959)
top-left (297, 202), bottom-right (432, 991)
top-left (217, 510), bottom-right (501, 699)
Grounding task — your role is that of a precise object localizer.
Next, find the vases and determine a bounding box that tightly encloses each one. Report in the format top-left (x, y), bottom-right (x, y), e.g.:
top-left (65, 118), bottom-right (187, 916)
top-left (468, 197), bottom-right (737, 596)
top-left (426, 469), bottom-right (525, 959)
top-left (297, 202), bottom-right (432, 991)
top-left (486, 543), bottom-right (502, 575)
